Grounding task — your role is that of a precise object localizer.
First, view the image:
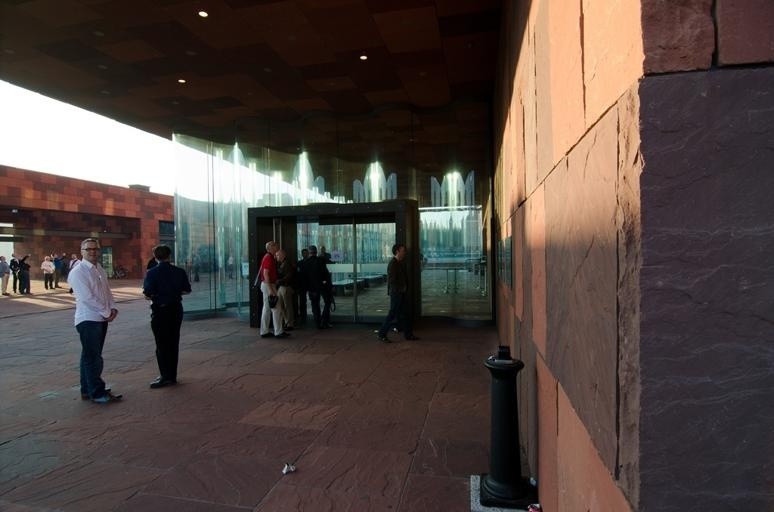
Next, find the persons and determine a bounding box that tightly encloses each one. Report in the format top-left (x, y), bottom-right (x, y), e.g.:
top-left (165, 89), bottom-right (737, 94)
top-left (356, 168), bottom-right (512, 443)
top-left (185, 241), bottom-right (336, 337)
top-left (143, 245), bottom-right (192, 389)
top-left (146, 246), bottom-right (158, 270)
top-left (0, 252), bottom-right (81, 293)
top-left (67, 237), bottom-right (122, 403)
top-left (377, 243), bottom-right (420, 343)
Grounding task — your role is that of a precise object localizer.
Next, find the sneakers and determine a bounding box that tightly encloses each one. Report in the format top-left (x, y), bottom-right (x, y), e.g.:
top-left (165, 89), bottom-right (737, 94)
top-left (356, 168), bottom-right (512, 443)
top-left (45, 284), bottom-right (61, 290)
top-left (283, 326), bottom-right (294, 330)
top-left (149, 373), bottom-right (176, 388)
top-left (275, 331), bottom-right (291, 338)
top-left (2, 292), bottom-right (10, 296)
top-left (14, 289), bottom-right (32, 296)
top-left (404, 334), bottom-right (418, 341)
top-left (80, 386), bottom-right (122, 403)
top-left (376, 332), bottom-right (390, 344)
top-left (262, 332), bottom-right (274, 339)
top-left (315, 319), bottom-right (333, 330)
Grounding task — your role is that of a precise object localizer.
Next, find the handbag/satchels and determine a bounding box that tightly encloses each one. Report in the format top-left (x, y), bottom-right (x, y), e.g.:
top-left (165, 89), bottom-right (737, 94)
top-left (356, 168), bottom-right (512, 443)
top-left (250, 280), bottom-right (259, 290)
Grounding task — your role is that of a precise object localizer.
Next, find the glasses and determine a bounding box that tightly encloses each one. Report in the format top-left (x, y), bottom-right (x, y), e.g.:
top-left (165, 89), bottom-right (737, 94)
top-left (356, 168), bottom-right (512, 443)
top-left (81, 247), bottom-right (101, 252)
